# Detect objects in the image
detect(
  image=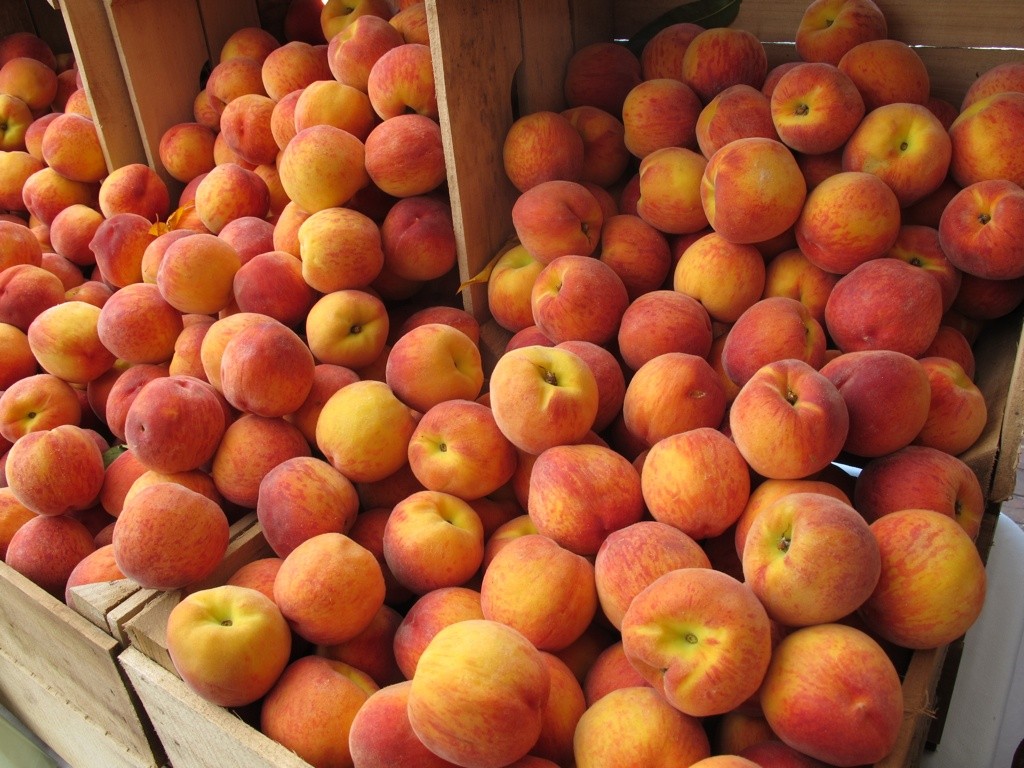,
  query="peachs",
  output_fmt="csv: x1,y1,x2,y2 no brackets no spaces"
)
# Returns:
0,0,1022,764
